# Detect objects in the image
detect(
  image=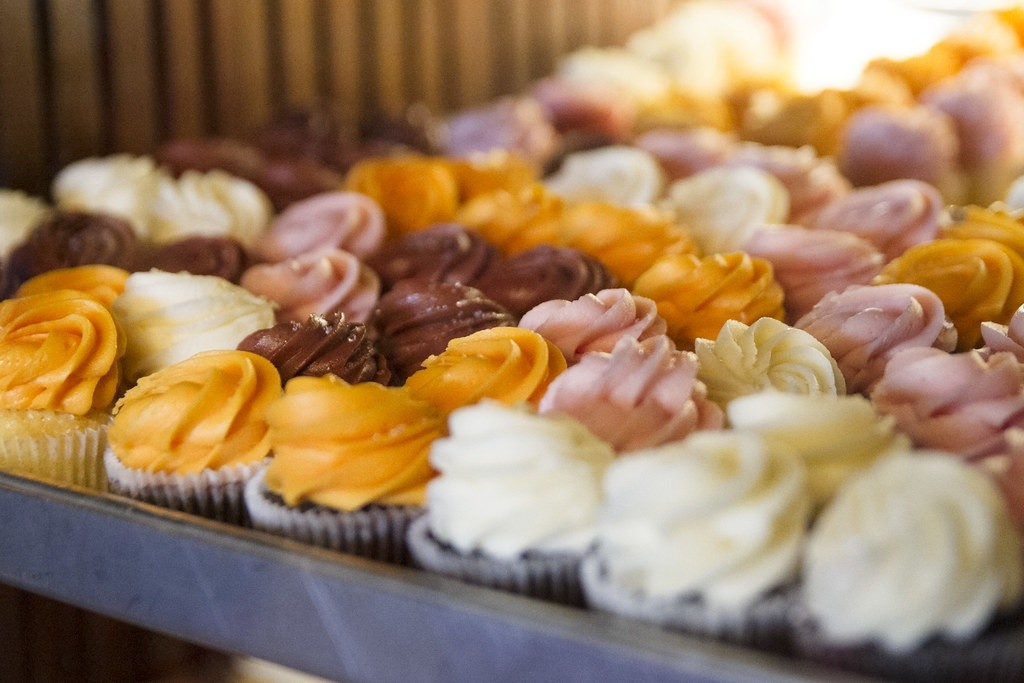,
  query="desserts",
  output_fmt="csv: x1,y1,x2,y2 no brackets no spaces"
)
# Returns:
0,0,1024,683
